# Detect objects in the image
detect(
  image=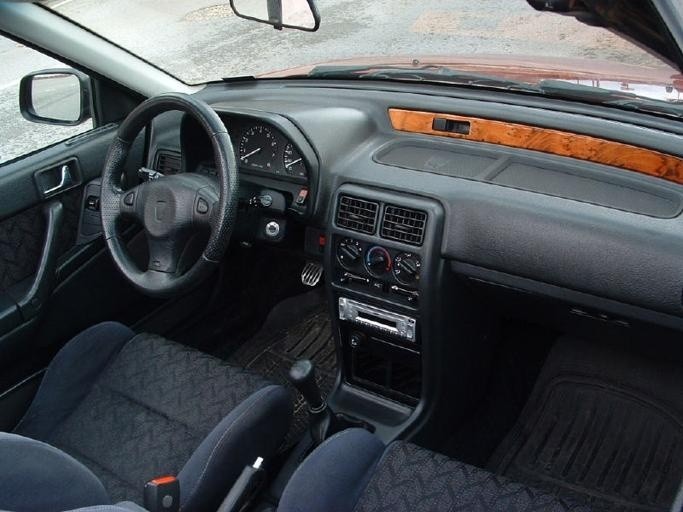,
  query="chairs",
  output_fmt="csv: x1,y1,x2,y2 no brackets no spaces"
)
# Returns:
4,324,299,508
275,427,588,507
